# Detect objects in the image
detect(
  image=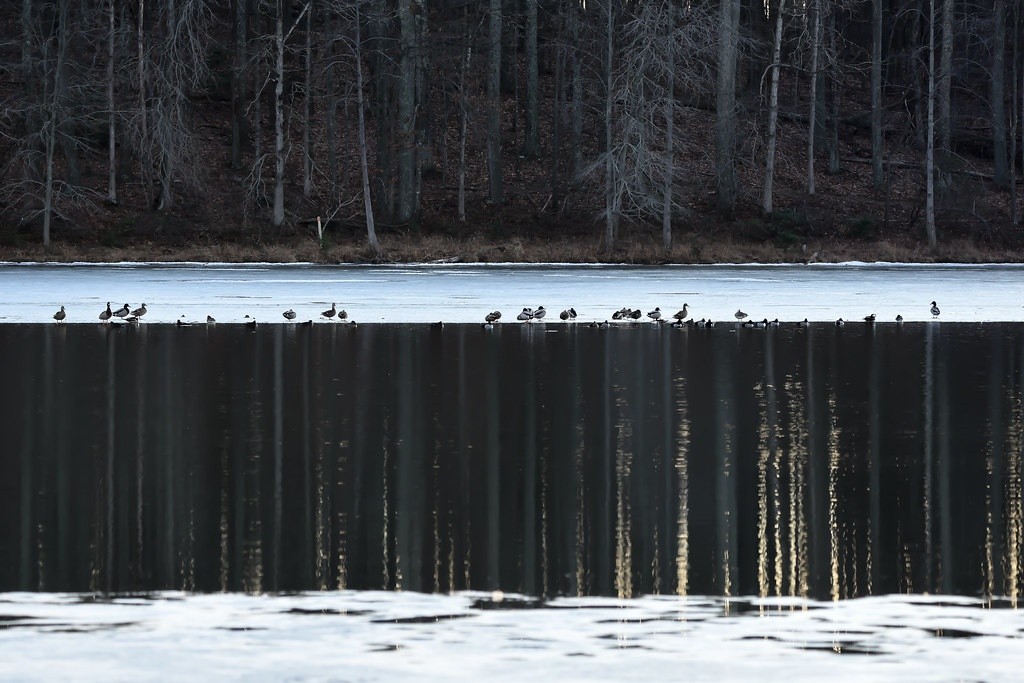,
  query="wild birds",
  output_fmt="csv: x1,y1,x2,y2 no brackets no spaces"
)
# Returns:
484,305,578,323
670,302,715,329
734,309,810,328
929,300,941,320
282,302,348,322
53,305,66,324
589,306,662,329
176,314,259,331
834,312,903,327
98,301,147,323
